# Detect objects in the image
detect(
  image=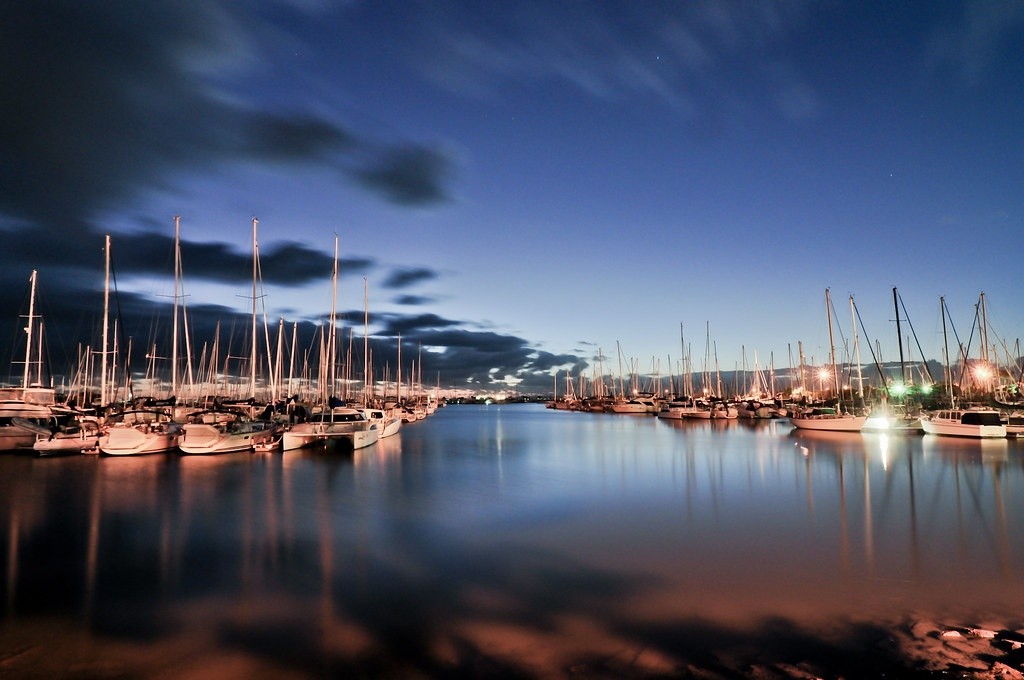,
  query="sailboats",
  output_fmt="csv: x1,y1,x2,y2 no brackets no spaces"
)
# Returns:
919,290,1008,438
1000,339,1024,437
0,269,71,452
31,235,121,452
788,289,923,432
98,214,446,455
544,321,793,421
